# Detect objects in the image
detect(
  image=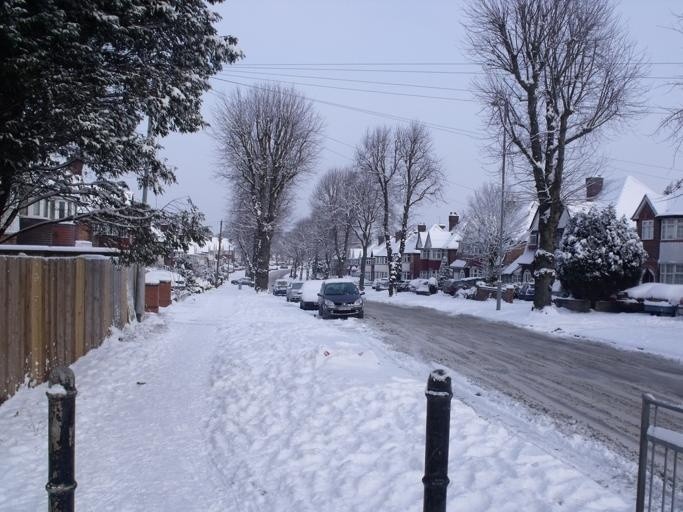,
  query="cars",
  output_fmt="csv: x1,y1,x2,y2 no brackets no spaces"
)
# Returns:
372,277,431,294
286,281,303,303
231,277,251,285
272,279,288,295
298,279,325,309
317,279,364,320
